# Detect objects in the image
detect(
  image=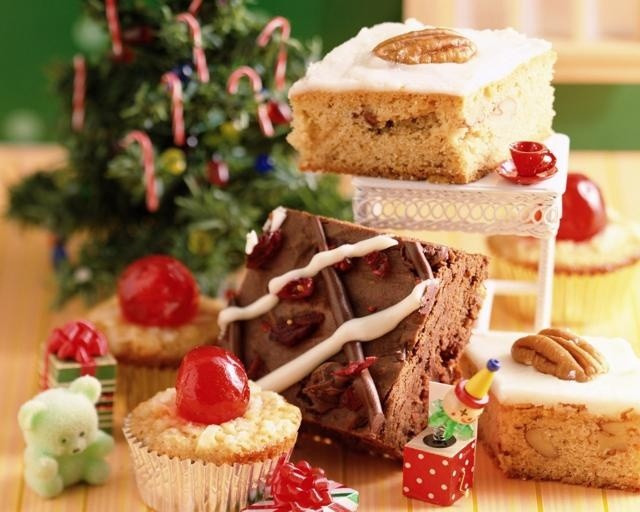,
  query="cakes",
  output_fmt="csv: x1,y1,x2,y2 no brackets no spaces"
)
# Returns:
122,346,301,512
284,19,556,185
91,255,229,416
459,329,640,493
216,205,489,458
488,173,640,325
